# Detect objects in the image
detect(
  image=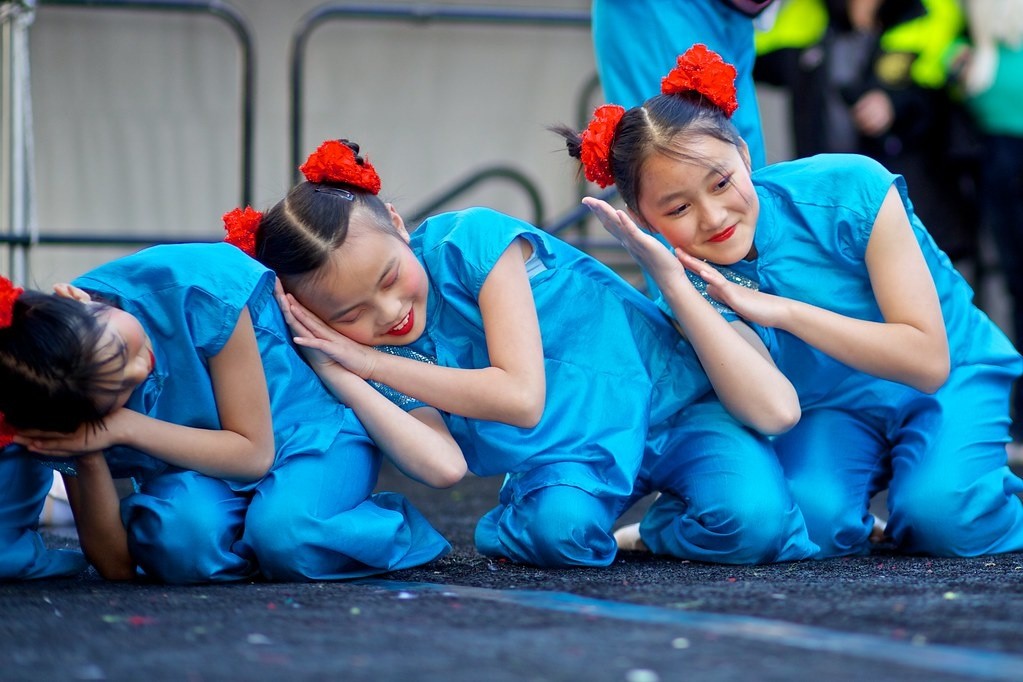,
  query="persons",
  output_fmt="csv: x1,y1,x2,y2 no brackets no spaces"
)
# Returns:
754,0,995,303
4,434,115,588
546,42,1021,560
585,0,772,176
214,141,825,566
962,29,1022,473
0,236,454,585
